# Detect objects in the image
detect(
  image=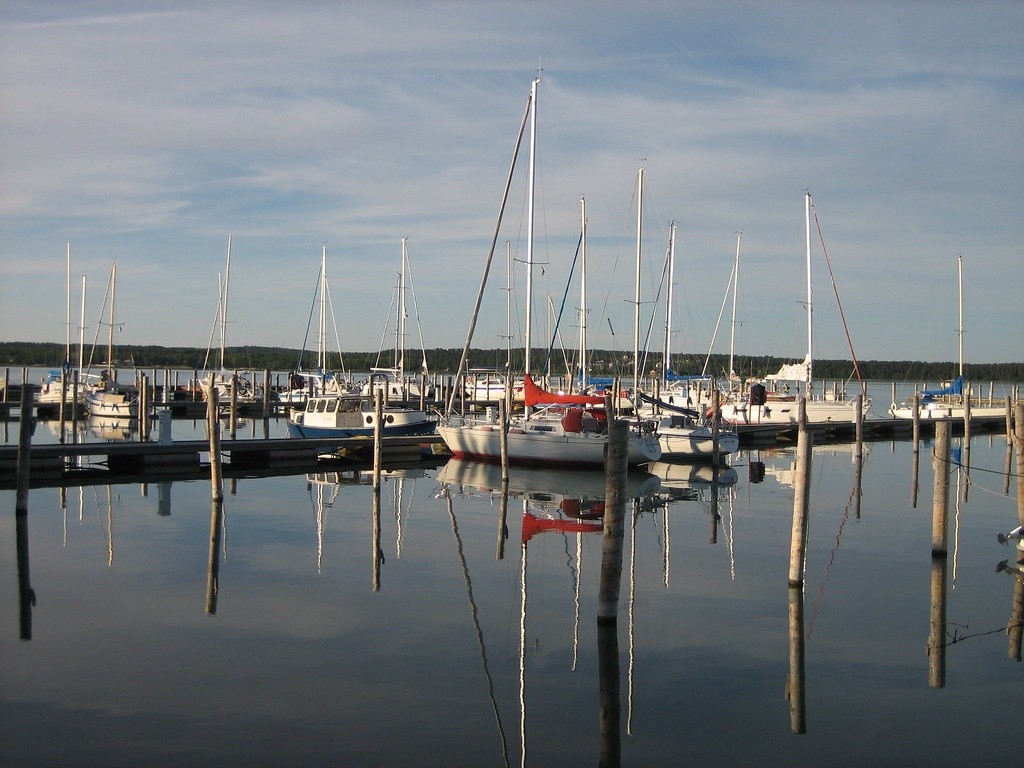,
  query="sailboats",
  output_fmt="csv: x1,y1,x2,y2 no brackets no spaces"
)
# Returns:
431,459,664,768
429,78,665,468
648,463,743,592
33,196,874,443
612,168,738,464
886,252,1014,416
